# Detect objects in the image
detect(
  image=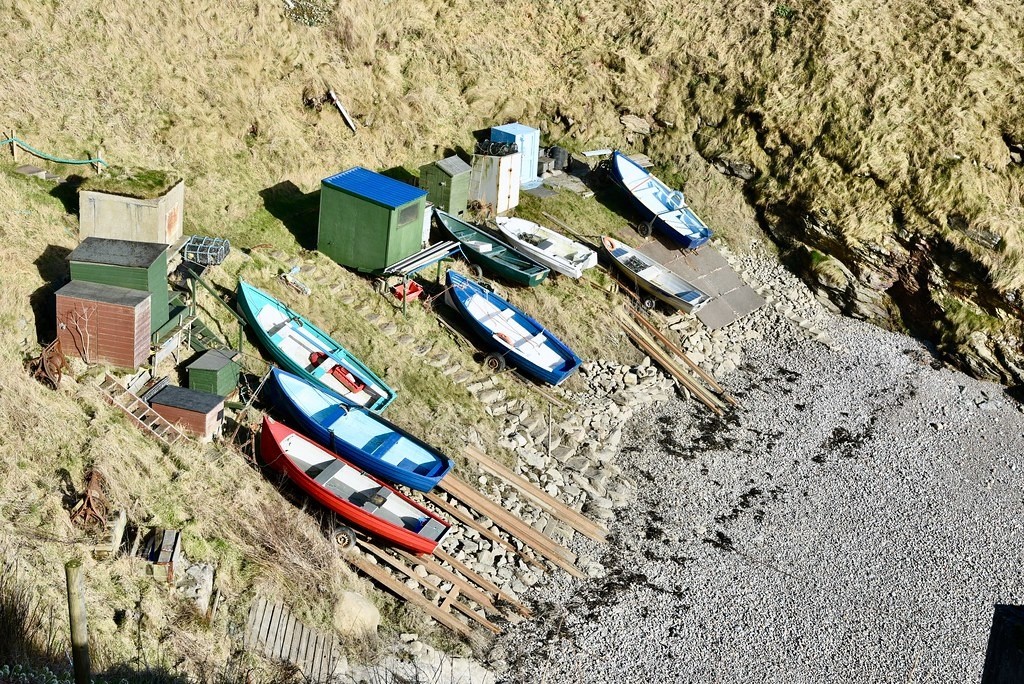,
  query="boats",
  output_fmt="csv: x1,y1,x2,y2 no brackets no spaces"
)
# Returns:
495,216,597,279
444,265,583,386
608,149,714,248
600,235,712,315
237,275,398,416
434,207,550,287
260,414,452,556
270,364,456,494
